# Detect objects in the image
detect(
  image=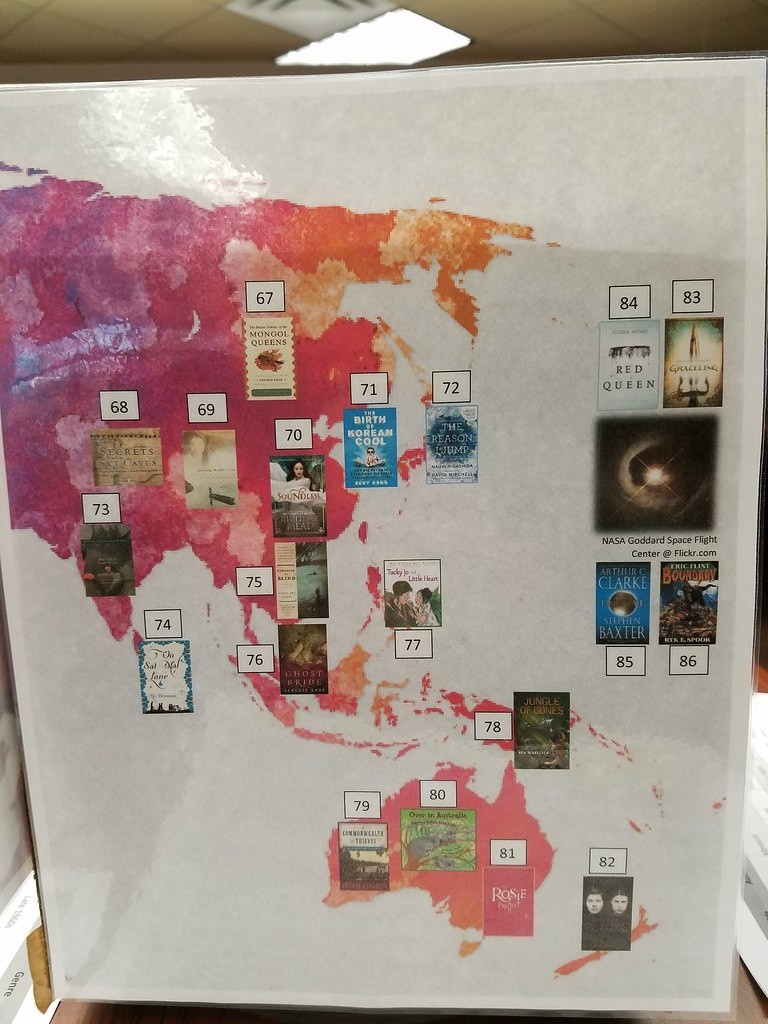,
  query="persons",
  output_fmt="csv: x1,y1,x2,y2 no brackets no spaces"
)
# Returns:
585,887,632,951
286,460,320,492
